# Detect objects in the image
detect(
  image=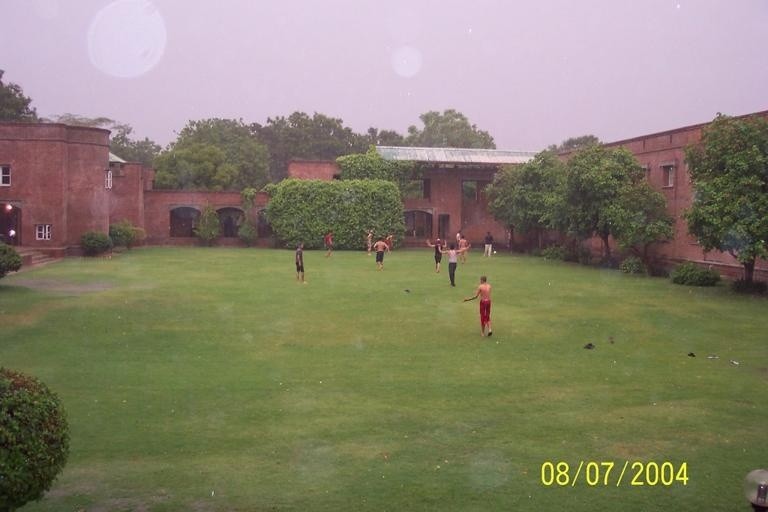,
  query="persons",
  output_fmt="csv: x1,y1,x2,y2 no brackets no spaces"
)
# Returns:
476,276,492,336
367,229,395,271
425,230,497,286
325,231,335,257
296,240,308,285
